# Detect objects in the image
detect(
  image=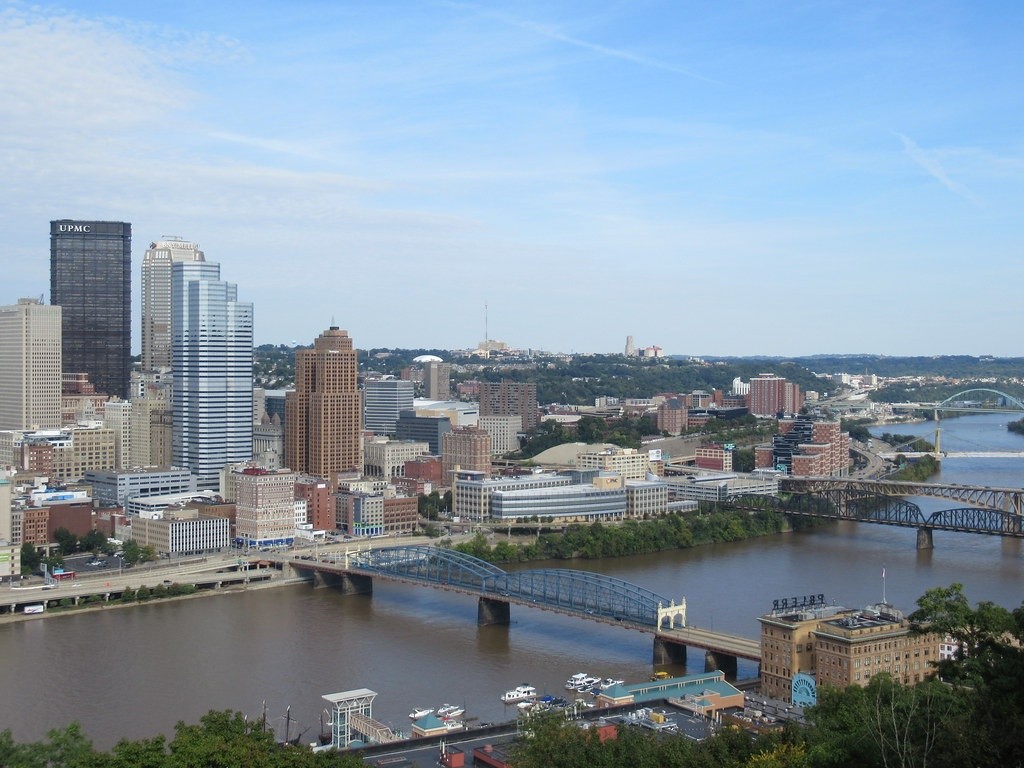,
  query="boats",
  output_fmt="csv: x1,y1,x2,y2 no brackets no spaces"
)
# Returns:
407,703,466,730
563,672,625,697
650,671,674,682
500,695,572,718
500,683,537,704
